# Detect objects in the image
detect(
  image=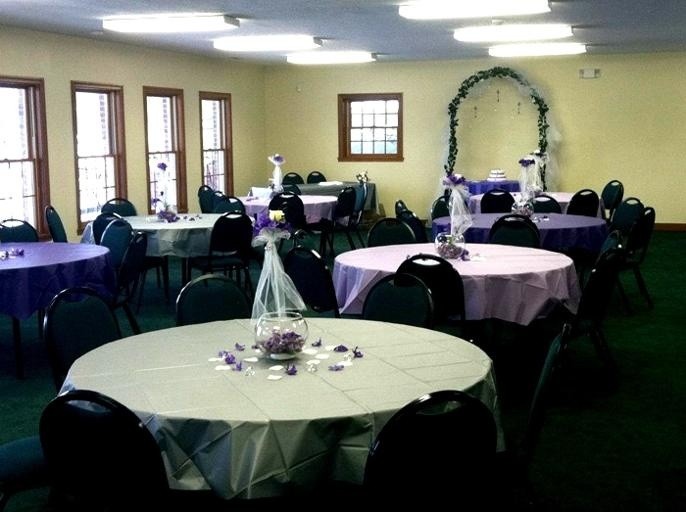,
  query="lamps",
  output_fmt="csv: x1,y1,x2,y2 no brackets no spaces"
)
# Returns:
214,34,321,55
454,22,576,44
101,13,240,35
287,50,378,64
399,0,554,21
488,42,589,59
